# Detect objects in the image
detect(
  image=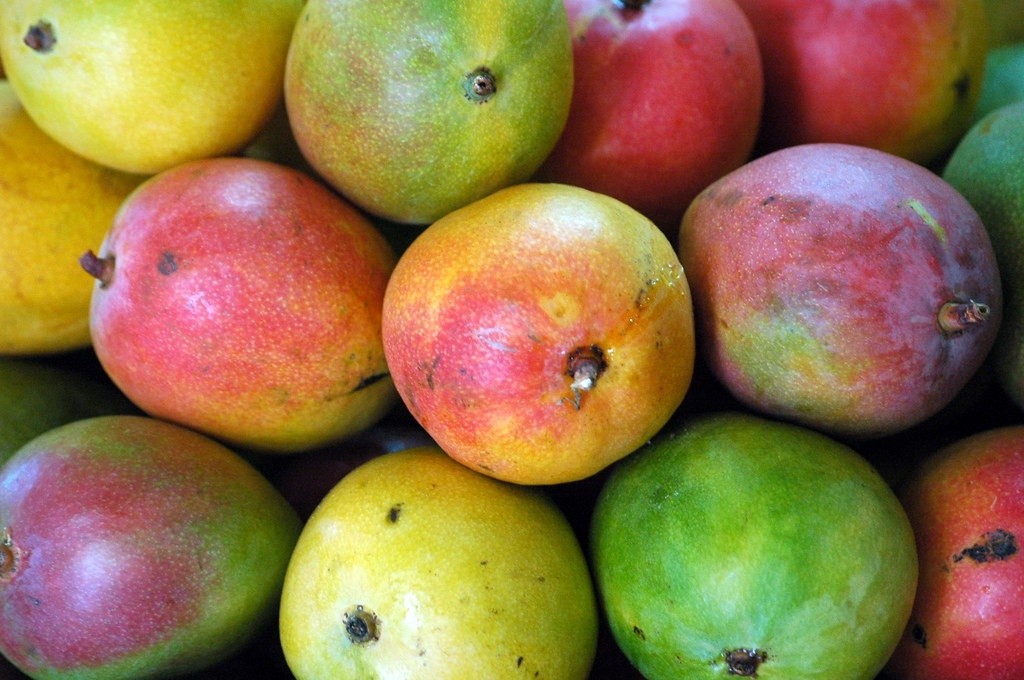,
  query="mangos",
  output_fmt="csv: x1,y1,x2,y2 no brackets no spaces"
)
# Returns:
0,1,1024,680
